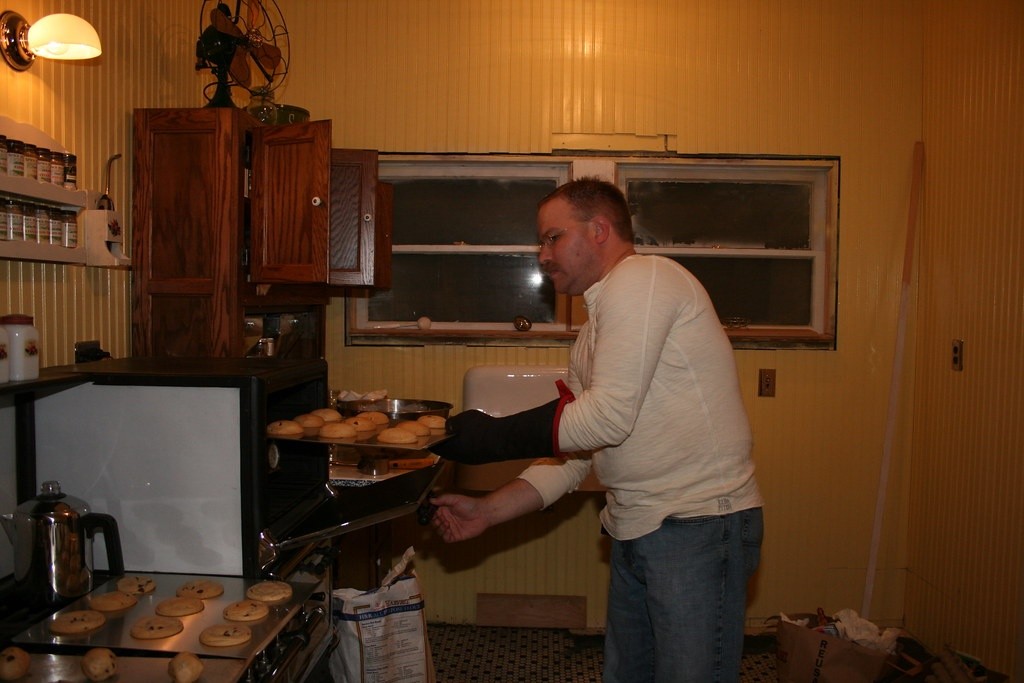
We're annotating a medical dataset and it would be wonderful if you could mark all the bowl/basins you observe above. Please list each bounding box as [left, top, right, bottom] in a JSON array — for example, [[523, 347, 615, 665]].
[[336, 398, 454, 427], [242, 104, 310, 125]]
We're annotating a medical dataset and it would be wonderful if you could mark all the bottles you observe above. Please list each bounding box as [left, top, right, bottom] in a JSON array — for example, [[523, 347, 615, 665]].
[[249, 85, 278, 125], [0, 313, 40, 384]]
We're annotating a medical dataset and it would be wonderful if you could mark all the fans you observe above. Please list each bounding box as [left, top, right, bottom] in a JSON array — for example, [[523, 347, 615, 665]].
[[195, 0, 290, 108]]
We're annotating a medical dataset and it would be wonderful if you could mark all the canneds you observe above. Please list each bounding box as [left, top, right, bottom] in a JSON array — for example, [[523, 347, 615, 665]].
[[0, 197, 78, 248], [0, 135, 77, 191]]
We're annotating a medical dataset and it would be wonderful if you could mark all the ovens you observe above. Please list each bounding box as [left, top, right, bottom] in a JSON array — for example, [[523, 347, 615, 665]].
[[14, 357, 447, 577]]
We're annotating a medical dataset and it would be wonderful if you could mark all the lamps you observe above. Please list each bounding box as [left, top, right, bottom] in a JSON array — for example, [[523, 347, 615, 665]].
[[0, 8, 102, 71]]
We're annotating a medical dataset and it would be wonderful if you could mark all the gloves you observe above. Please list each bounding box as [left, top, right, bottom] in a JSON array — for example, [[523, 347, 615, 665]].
[[428, 379, 576, 467]]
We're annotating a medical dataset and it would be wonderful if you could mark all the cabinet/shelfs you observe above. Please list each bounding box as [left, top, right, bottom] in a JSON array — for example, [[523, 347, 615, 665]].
[[132, 104, 393, 359], [0, 117, 85, 265]]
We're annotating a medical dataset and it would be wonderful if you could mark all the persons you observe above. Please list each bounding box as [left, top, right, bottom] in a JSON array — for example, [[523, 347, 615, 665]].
[[430, 175, 764, 683]]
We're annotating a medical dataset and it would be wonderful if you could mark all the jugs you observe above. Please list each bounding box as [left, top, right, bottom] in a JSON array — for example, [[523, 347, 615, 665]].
[[0, 480, 126, 606]]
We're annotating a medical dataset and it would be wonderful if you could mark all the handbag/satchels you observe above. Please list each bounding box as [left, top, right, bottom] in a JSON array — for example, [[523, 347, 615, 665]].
[[762, 611, 888, 683]]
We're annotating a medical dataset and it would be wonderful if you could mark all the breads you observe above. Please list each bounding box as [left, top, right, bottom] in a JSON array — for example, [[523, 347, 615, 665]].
[[265, 407, 448, 445]]
